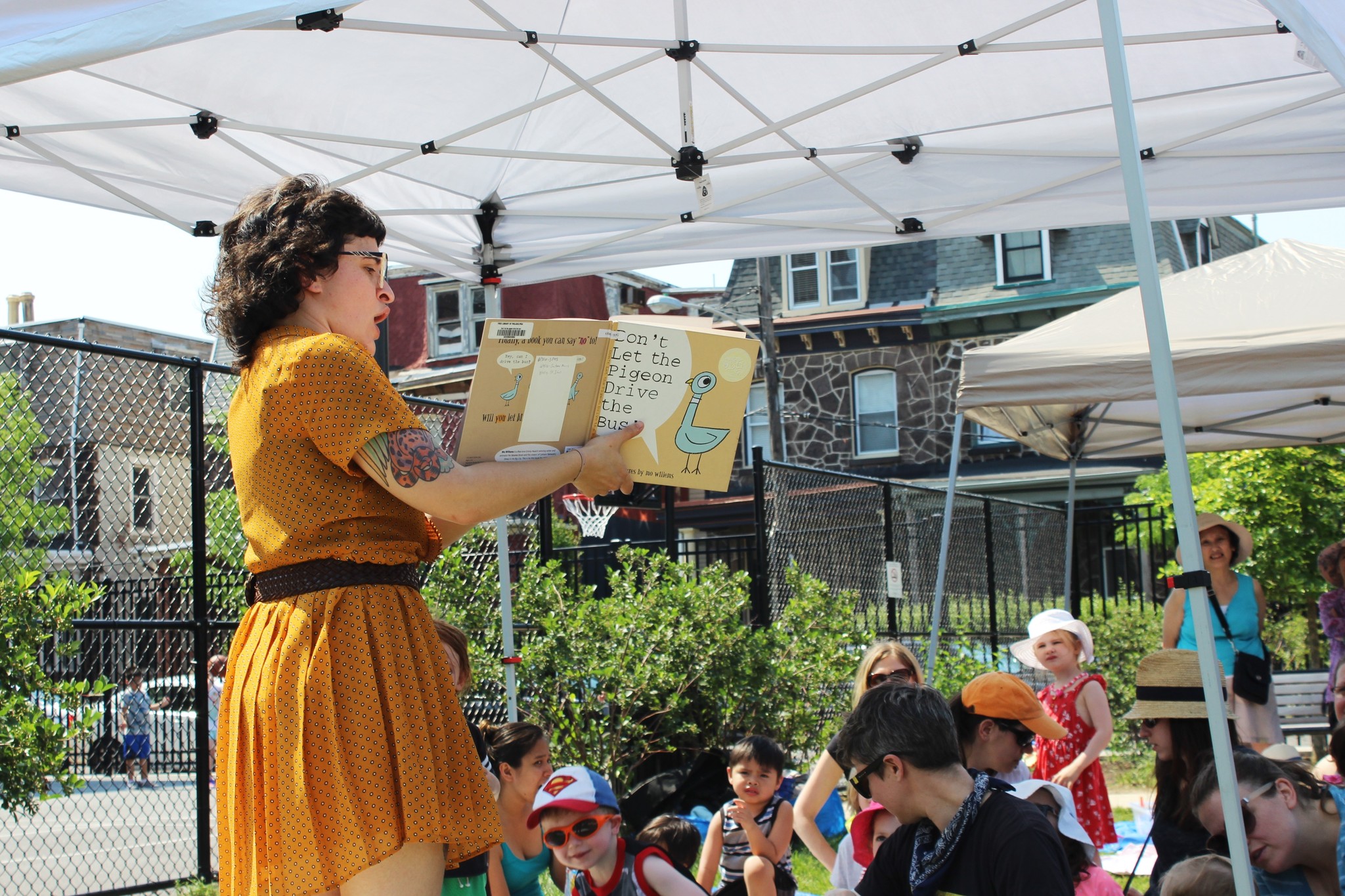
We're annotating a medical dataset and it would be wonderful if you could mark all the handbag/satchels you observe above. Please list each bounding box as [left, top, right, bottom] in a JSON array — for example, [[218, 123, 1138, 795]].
[[1233, 649, 1271, 706]]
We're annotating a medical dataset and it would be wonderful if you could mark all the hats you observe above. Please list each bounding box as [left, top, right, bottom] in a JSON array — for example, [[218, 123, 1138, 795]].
[[850, 800, 885, 869], [1009, 609, 1094, 670], [960, 671, 1069, 740], [526, 765, 620, 830], [1262, 742, 1305, 771], [1175, 512, 1252, 568], [1122, 649, 1240, 720]]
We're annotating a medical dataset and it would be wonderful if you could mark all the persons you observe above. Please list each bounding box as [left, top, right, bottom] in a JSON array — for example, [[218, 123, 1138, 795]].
[[1308, 536, 1345, 788], [1190, 752, 1345, 896], [1160, 511, 1288, 759], [522, 642, 1126, 896], [1118, 648, 1269, 896], [120, 667, 171, 789], [201, 174, 644, 896], [1006, 609, 1119, 872], [207, 670, 225, 791]]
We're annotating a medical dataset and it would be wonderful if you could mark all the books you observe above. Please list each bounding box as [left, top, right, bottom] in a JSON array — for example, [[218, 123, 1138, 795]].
[[455, 314, 761, 492]]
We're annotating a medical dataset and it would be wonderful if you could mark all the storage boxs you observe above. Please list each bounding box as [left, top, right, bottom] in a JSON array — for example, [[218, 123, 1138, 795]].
[[1133, 793, 1154, 835]]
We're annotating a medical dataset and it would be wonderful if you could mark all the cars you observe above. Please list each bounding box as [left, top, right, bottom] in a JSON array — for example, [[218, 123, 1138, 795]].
[[14, 672, 195, 777]]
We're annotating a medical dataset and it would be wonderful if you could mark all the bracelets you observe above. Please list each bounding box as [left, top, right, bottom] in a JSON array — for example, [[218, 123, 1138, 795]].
[[572, 449, 585, 484]]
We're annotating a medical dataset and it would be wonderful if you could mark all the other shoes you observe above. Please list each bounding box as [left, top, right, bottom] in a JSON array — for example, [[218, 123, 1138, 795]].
[[128, 780, 155, 788]]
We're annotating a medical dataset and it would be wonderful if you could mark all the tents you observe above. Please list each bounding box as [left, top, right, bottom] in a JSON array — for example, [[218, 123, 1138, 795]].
[[922, 244, 1345, 700], [0, 0, 1345, 896]]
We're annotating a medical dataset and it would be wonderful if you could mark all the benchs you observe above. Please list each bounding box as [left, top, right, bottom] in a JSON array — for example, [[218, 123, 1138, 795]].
[[1273, 674, 1334, 758]]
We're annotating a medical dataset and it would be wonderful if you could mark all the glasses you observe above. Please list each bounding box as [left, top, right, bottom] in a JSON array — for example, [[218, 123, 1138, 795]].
[[1143, 718, 1159, 728], [332, 252, 387, 289], [1205, 781, 1278, 858], [543, 814, 615, 849], [988, 719, 1035, 748], [867, 668, 914, 688], [848, 751, 911, 799]]
